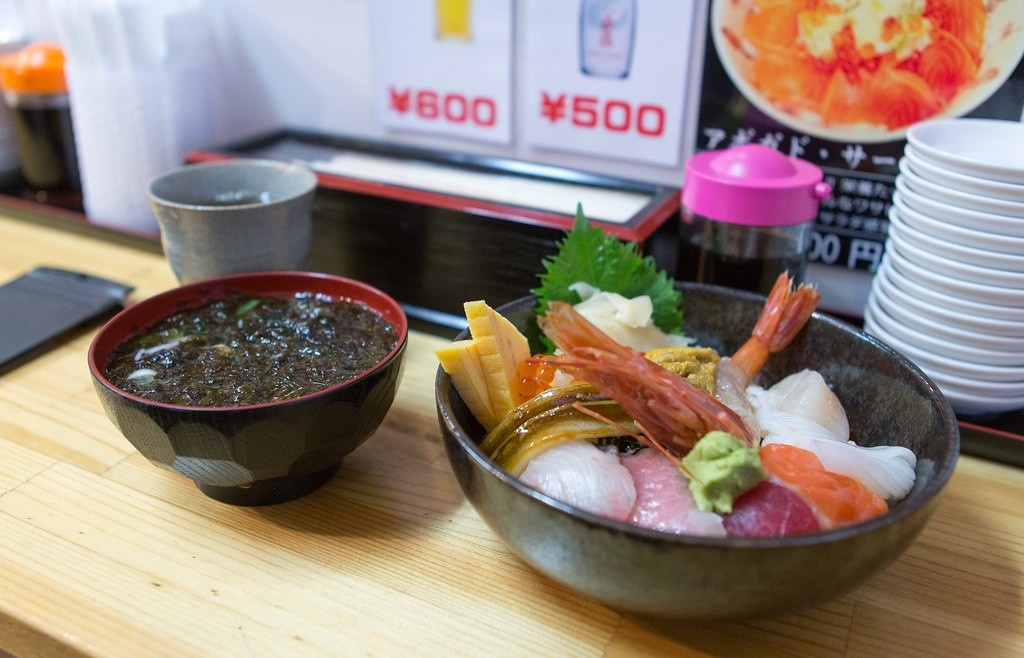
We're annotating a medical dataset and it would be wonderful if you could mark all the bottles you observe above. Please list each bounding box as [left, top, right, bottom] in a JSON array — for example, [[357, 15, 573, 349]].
[[674, 143, 832, 296], [579, 0, 636, 79]]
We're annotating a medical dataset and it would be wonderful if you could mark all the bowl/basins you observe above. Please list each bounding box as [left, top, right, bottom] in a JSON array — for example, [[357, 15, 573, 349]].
[[87, 272, 408, 506], [435, 281, 961, 624]]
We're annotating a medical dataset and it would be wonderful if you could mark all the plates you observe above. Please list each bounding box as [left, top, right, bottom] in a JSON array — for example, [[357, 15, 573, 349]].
[[711, 0, 1023, 144], [863, 119, 1024, 416]]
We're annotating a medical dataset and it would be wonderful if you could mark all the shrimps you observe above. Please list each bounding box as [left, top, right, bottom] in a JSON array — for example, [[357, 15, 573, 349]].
[[526, 269, 820, 457]]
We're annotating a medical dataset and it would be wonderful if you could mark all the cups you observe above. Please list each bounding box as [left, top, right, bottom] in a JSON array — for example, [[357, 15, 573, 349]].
[[145, 159, 319, 285]]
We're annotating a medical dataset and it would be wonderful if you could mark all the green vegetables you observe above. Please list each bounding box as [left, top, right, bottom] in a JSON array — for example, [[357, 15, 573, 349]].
[[526, 207, 685, 353]]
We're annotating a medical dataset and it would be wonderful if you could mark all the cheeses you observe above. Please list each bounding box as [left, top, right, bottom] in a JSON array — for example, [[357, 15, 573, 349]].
[[437, 299, 532, 431]]
